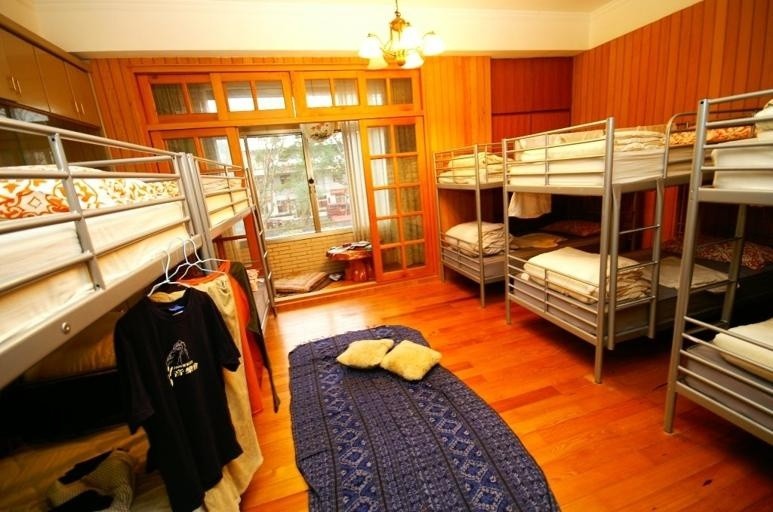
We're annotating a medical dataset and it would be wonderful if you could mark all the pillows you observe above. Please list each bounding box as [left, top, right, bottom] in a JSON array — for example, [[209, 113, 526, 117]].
[[335, 338, 442, 381]]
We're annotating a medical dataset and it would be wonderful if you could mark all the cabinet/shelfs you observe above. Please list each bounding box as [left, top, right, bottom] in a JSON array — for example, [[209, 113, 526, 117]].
[[0, 12, 106, 132]]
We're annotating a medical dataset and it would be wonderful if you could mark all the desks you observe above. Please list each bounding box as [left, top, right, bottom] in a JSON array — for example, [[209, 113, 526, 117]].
[[326, 241, 397, 282]]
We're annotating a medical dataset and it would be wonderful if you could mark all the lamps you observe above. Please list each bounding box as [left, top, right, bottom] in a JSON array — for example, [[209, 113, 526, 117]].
[[360, 0, 445, 69]]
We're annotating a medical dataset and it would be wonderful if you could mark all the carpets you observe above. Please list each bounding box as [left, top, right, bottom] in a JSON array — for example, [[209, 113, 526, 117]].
[[288, 325, 562, 512]]
